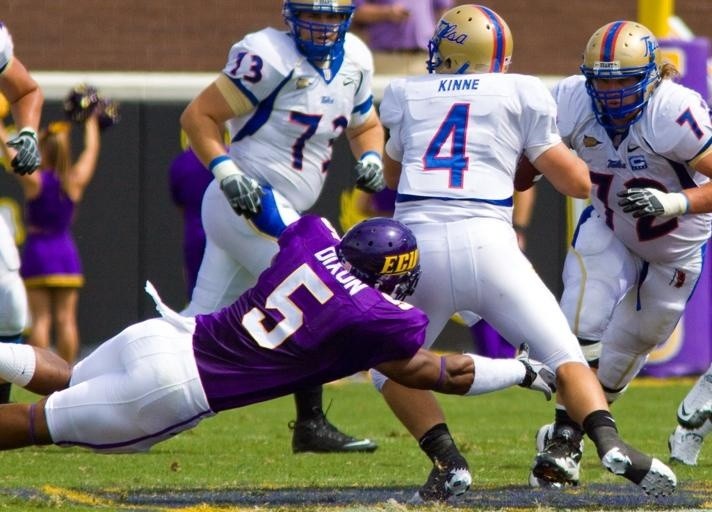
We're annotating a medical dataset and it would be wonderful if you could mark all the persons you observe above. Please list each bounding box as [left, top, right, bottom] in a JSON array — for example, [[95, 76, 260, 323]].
[[352, 0, 454, 55], [0, 24, 45, 407], [1, 186, 476, 451], [168, 126, 233, 306], [668, 363, 712, 467], [180, 2, 380, 453], [370, 4, 678, 505], [514, 20, 712, 490], [1, 113, 100, 369]]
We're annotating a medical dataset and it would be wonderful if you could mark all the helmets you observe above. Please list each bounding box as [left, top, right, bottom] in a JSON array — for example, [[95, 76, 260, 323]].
[[335, 217, 422, 302], [426, 2, 514, 74], [282, 0, 355, 61], [580, 20, 660, 129]]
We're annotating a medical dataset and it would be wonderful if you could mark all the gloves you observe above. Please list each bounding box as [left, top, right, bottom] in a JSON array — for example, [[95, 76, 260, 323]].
[[515, 342, 556, 401], [617, 188, 686, 218], [209, 156, 265, 218], [355, 152, 385, 192], [6, 131, 39, 175]]
